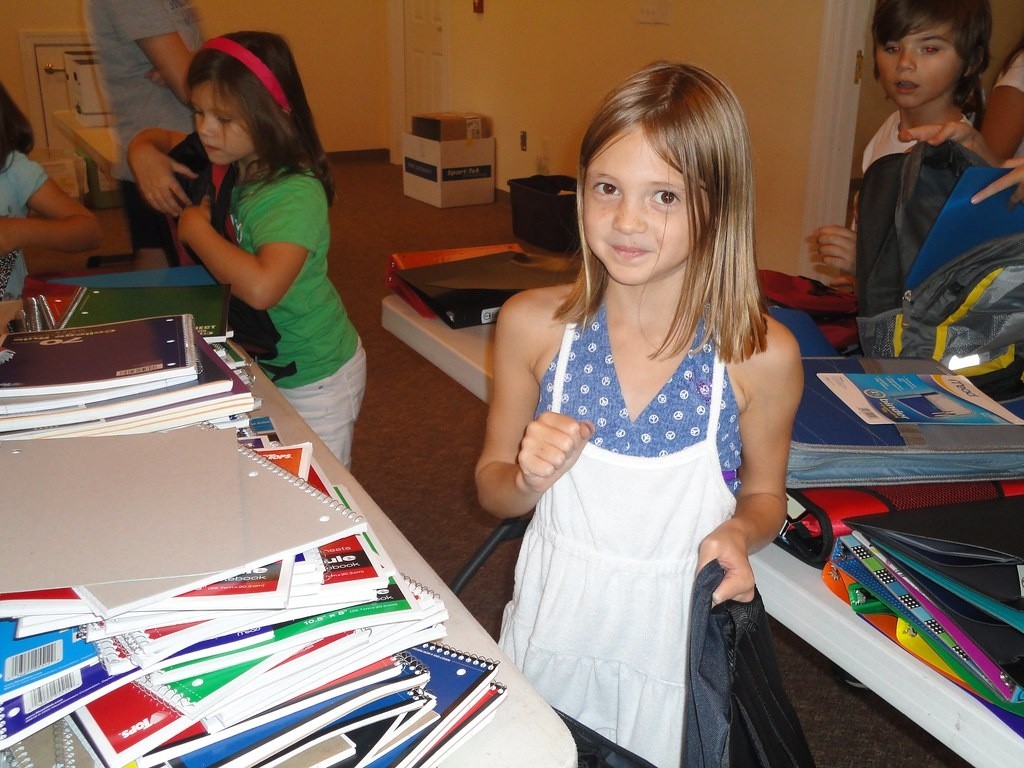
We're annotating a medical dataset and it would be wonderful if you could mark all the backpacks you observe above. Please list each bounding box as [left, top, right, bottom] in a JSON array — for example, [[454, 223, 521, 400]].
[[682, 561, 814, 768], [855, 139, 1024, 379], [163, 131, 298, 382]]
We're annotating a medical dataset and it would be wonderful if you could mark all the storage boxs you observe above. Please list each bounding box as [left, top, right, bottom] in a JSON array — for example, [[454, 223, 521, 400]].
[[408, 109, 494, 145], [62, 49, 118, 127], [400, 129, 496, 209], [506, 176, 580, 252]]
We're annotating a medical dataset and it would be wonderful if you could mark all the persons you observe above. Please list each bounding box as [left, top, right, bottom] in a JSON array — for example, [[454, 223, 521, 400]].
[[475, 60, 804, 768], [895, 39, 1023, 211], [0, 81, 105, 302], [816, 0, 995, 295], [86, 0, 205, 252], [128, 31, 367, 472]]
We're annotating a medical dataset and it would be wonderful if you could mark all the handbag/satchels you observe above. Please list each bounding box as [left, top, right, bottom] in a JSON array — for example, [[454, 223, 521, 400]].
[[757, 268, 859, 357]]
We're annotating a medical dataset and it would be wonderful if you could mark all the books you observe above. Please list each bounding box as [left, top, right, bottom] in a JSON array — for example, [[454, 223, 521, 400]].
[[0, 283, 507, 768]]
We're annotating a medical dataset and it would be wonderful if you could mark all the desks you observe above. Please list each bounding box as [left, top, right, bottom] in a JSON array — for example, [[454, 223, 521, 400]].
[[2, 339, 577, 768], [49, 110, 136, 266], [379, 293, 1024, 768]]
